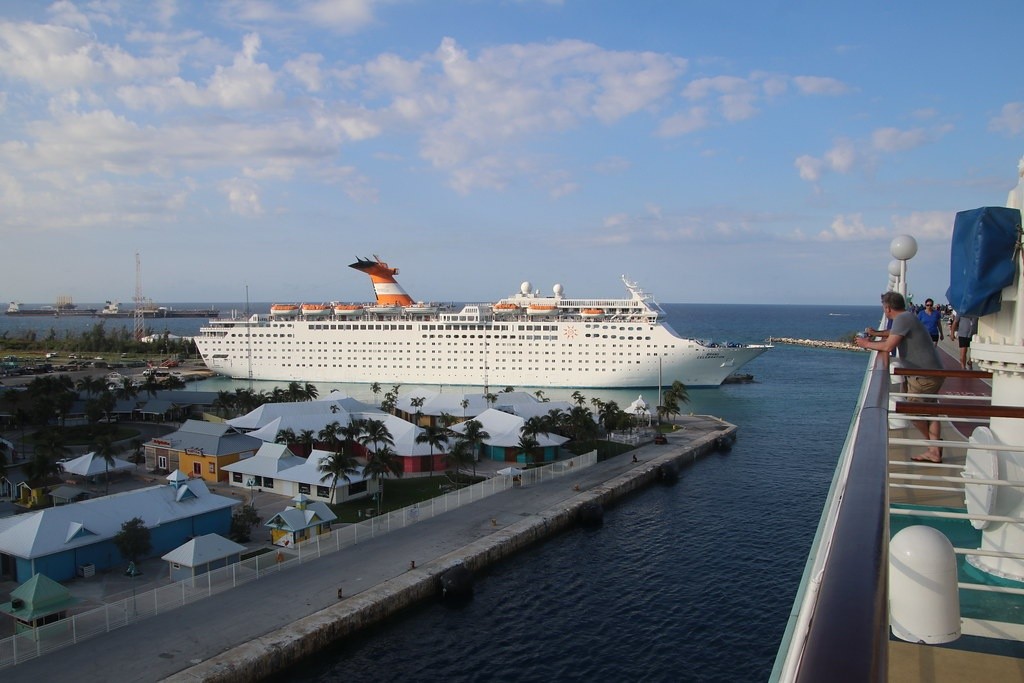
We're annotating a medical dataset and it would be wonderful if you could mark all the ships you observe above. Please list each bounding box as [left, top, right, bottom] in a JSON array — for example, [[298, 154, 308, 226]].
[[190, 253, 775, 389]]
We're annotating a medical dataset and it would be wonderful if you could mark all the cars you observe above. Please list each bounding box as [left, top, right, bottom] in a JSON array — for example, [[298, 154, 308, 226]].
[[59, 352, 133, 370]]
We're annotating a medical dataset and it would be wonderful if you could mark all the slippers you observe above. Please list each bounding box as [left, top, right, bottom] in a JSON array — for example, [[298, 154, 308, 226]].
[[911, 455, 942, 463]]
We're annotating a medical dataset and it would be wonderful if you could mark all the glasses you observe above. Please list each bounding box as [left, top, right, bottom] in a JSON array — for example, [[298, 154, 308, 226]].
[[926, 304, 933, 307]]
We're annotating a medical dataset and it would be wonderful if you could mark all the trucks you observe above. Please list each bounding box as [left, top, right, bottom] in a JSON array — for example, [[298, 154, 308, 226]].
[[143, 366, 171, 378]]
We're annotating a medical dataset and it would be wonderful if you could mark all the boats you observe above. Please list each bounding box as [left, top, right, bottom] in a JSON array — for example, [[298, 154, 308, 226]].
[[270, 303, 300, 317], [404, 301, 439, 315], [141, 333, 183, 345], [301, 303, 332, 316], [368, 302, 403, 316], [491, 303, 522, 317], [334, 304, 363, 316], [525, 303, 559, 316], [580, 308, 605, 319]]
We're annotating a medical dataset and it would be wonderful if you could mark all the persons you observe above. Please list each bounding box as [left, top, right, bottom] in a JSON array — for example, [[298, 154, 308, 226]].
[[633, 454, 637, 461], [854, 292, 948, 463], [909, 298, 978, 370]]
